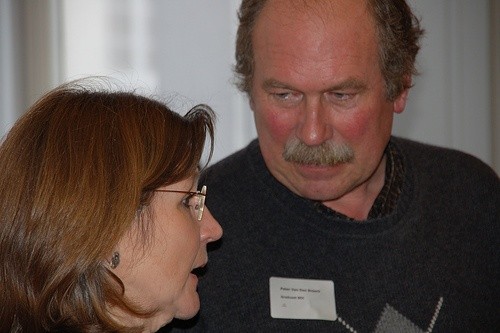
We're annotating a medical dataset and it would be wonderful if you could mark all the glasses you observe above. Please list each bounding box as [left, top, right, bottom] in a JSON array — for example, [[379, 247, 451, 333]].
[[148, 186, 207, 222]]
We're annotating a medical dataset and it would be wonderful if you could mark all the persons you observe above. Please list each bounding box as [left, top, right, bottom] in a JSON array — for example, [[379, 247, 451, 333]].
[[157, 0, 500, 332], [1, 76, 223, 332]]
